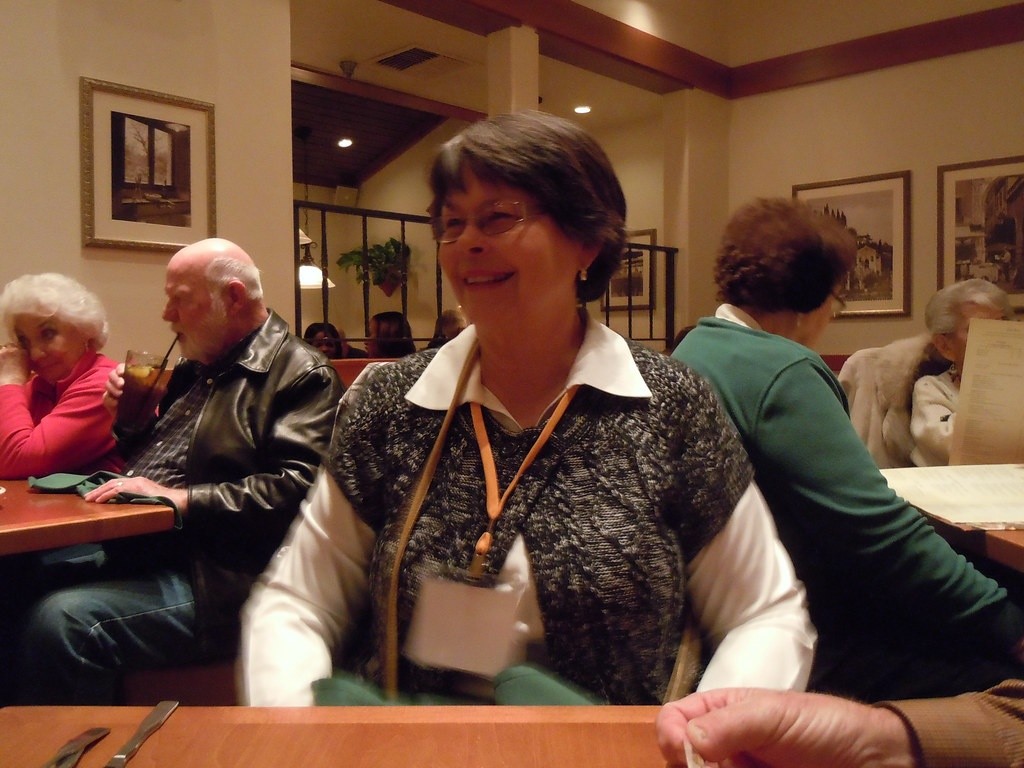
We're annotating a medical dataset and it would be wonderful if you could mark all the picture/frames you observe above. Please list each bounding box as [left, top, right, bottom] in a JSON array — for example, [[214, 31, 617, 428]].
[[936, 155, 1024, 315], [79, 75, 217, 252], [600, 229, 656, 311], [791, 169, 913, 320]]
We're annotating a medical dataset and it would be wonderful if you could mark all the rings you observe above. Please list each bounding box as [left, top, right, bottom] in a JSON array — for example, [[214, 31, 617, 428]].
[[2, 346, 6, 349], [118, 481, 123, 486]]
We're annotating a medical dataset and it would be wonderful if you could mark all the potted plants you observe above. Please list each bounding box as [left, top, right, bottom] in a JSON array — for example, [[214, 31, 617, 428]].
[[336, 238, 411, 298]]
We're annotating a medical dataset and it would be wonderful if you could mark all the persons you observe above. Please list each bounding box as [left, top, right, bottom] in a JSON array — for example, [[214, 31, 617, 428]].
[[241, 109, 817, 704], [659, 679, 1023, 768], [302, 322, 343, 359], [365, 310, 418, 358], [1, 271, 131, 479], [907, 278, 1024, 464], [0, 238, 346, 705], [663, 196, 1024, 701], [337, 326, 369, 358], [427, 309, 468, 349]]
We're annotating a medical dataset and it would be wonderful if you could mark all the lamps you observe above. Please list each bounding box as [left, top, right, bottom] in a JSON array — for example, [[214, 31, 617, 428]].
[[297, 124, 337, 289]]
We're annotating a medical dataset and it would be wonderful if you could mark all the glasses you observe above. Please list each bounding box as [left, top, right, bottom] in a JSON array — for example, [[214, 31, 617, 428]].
[[430, 197, 543, 244], [826, 284, 849, 319]]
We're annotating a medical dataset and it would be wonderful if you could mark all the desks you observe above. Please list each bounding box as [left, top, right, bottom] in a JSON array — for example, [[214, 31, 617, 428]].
[[0, 705, 676, 767], [0, 476, 174, 557]]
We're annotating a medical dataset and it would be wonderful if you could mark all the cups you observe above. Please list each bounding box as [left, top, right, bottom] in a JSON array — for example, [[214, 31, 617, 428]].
[[113, 350, 177, 445]]
